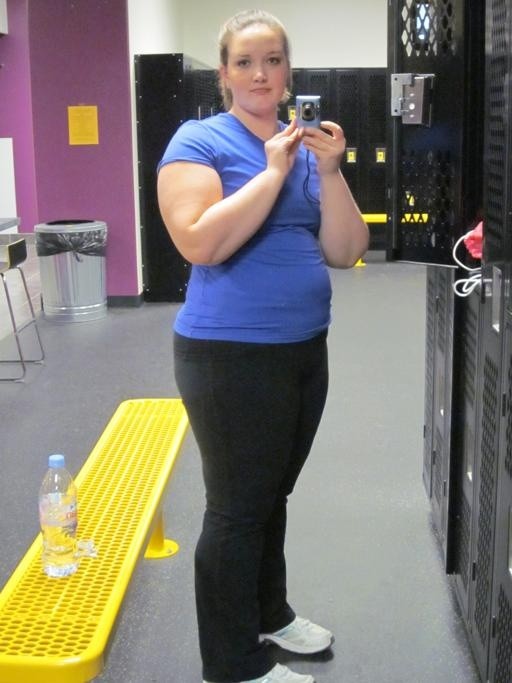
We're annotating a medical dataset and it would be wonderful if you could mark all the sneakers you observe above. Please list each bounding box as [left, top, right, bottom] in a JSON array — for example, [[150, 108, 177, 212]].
[[202, 661, 315, 682], [259, 615, 336, 653]]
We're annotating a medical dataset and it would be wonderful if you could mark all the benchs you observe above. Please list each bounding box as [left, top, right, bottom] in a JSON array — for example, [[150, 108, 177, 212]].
[[1, 396, 191, 682]]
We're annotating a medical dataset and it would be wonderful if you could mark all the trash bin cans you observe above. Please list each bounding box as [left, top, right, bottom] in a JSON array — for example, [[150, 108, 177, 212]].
[[33, 219, 107, 323]]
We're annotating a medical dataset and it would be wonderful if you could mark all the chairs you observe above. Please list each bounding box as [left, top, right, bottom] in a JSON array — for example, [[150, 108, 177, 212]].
[[0, 236, 45, 383]]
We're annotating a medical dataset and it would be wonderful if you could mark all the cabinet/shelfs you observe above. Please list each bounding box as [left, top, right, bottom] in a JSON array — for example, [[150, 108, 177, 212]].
[[134, 53, 390, 304], [377, 1, 512, 683]]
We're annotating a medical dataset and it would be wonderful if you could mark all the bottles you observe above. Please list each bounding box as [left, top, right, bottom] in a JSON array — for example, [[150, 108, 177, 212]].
[[37, 453, 78, 583]]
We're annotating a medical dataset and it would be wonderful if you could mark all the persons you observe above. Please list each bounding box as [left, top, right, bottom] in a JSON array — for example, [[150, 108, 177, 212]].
[[157, 10, 373, 682]]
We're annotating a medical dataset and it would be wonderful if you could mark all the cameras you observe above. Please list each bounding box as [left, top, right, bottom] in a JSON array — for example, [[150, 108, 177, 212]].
[[295, 94, 321, 134]]
